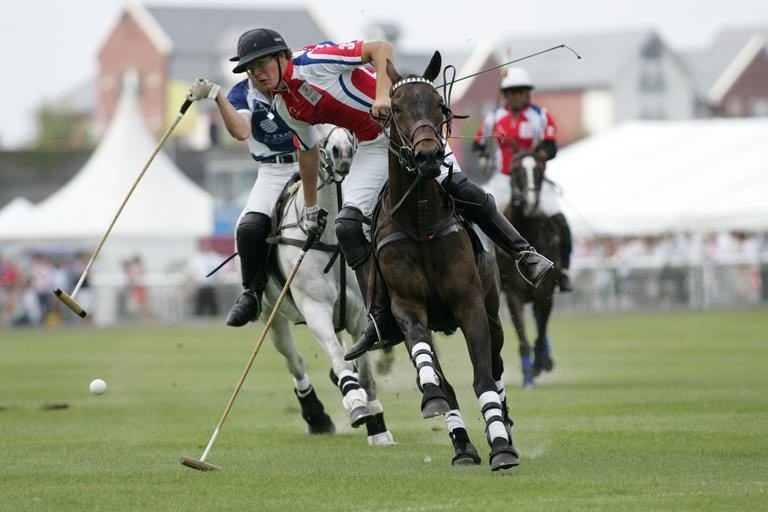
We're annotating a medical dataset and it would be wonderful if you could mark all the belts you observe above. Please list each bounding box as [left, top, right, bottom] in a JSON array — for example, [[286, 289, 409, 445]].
[[261, 152, 298, 163]]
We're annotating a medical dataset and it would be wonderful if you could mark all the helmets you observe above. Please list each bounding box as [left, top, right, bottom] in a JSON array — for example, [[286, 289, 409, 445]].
[[499, 66, 534, 91], [232, 30, 287, 73], [230, 28, 269, 64]]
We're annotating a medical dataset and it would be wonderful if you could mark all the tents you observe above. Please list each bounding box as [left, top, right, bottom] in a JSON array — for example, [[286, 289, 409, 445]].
[[0, 68, 216, 325]]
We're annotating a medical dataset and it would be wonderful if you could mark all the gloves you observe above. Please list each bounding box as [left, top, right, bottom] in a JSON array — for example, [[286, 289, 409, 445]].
[[301, 206, 327, 242], [187, 77, 221, 102]]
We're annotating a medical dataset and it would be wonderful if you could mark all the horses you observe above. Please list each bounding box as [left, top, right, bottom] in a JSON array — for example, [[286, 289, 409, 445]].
[[370, 51, 520, 471], [494, 136, 564, 392], [234, 123, 395, 444]]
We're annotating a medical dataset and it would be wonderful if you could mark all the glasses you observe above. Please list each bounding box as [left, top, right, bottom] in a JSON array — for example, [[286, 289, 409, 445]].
[[246, 57, 275, 73]]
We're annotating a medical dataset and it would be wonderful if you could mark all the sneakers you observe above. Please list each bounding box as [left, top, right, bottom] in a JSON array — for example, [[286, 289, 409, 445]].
[[527, 249, 550, 283], [343, 311, 394, 361], [558, 275, 574, 293], [226, 291, 261, 326]]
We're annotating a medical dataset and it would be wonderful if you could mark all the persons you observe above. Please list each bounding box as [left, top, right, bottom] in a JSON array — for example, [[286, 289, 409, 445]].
[[1, 237, 224, 332], [467, 63, 577, 297], [231, 28, 557, 363], [570, 225, 765, 308], [184, 27, 302, 330]]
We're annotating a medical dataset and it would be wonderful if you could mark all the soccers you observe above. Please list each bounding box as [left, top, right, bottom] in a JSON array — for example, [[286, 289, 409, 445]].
[[90, 380, 105, 395]]
